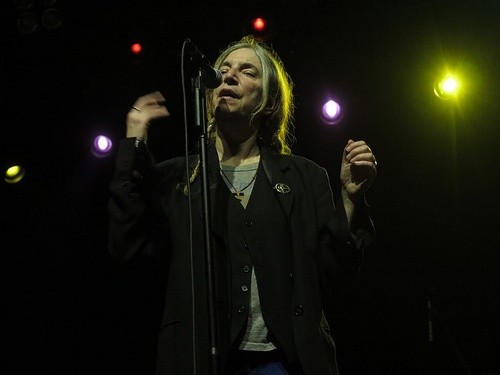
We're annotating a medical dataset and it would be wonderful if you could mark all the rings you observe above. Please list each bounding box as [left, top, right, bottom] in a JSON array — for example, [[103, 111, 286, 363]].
[[373, 161, 378, 168], [131, 104, 141, 113]]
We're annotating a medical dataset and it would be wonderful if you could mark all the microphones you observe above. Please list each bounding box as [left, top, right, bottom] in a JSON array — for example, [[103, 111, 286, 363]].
[[185, 38, 223, 89]]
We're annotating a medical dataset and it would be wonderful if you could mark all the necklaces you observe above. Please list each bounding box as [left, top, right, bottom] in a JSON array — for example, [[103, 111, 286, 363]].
[[218, 167, 258, 200]]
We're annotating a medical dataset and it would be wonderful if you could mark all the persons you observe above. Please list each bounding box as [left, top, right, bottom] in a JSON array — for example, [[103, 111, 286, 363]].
[[105, 37, 380, 375]]
